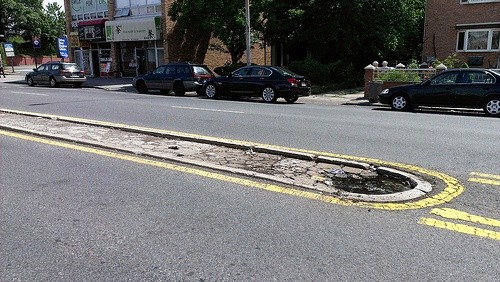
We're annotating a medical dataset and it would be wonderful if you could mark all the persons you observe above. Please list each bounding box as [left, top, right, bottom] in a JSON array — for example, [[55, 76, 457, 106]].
[[0, 58, 6, 78]]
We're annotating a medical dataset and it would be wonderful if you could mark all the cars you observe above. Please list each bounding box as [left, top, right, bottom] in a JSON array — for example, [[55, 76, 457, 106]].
[[131, 63, 221, 96], [378, 67, 500, 118], [26, 61, 87, 88], [195, 64, 312, 103]]
